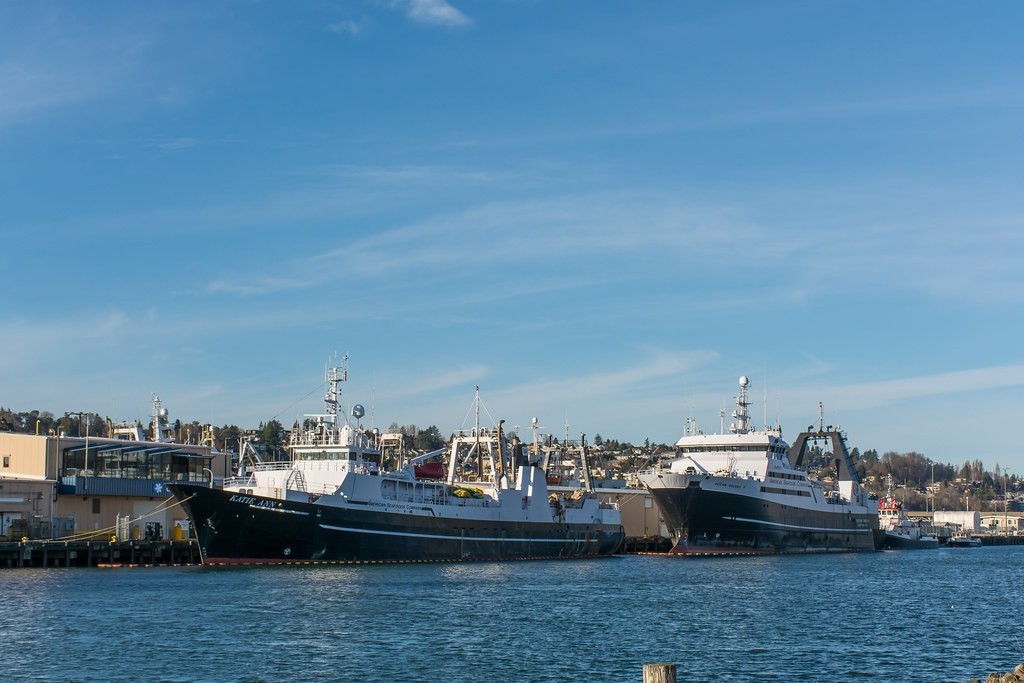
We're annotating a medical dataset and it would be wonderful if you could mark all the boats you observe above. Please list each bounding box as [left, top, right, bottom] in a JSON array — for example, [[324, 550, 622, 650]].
[[882, 525, 940, 550], [945, 534, 983, 548], [636, 363, 886, 555], [159, 349, 631, 568]]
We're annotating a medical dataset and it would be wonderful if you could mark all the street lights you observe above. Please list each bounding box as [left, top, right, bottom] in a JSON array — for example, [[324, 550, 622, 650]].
[[1000, 465, 1012, 537], [928, 460, 938, 525]]
